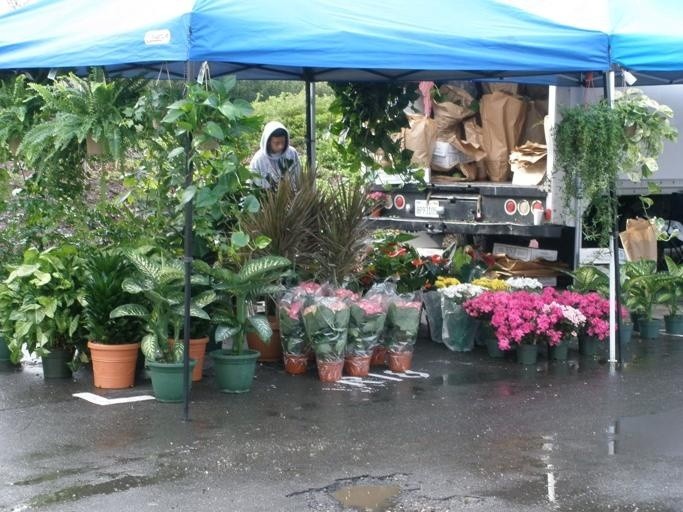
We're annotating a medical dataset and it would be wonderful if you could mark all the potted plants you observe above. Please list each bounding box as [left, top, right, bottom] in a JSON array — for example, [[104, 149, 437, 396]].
[[0, 68, 283, 403], [554, 86, 677, 242], [559, 255, 683, 339]]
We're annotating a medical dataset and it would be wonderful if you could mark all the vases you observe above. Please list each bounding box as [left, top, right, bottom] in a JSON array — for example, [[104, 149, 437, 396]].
[[283, 345, 413, 381], [486, 322, 634, 366]]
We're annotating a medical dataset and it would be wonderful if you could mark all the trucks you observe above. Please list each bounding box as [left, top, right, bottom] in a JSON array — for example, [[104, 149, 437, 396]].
[[352, 80, 683, 286]]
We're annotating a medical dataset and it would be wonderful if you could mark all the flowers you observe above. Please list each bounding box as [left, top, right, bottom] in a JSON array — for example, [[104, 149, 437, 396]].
[[283, 234, 629, 351], [417, 80, 479, 118]]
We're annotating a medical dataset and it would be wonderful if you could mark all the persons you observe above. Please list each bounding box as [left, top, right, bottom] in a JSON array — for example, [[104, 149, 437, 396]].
[[245, 121, 303, 197]]
